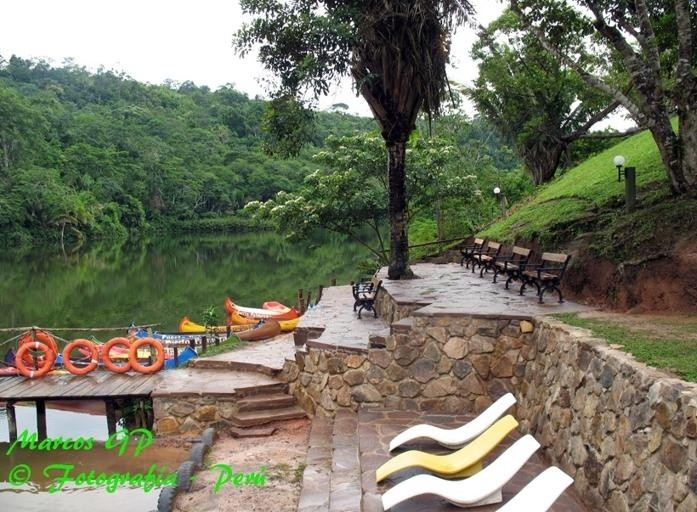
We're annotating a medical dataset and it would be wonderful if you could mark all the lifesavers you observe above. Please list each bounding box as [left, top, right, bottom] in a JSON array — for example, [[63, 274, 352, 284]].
[[15, 332, 57, 377], [63, 338, 99, 374], [130, 337, 165, 373], [103, 338, 132, 373]]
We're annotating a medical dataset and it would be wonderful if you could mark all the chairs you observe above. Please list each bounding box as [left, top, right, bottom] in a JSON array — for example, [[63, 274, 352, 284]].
[[460, 238, 571, 303], [352, 276, 383, 319], [376, 392, 574, 512]]
